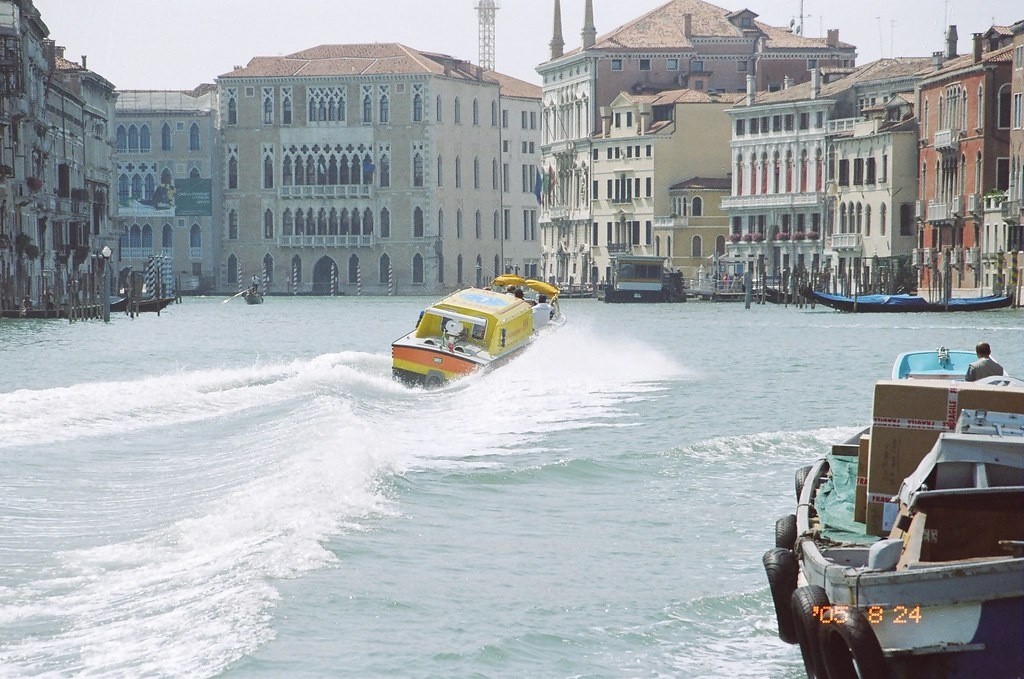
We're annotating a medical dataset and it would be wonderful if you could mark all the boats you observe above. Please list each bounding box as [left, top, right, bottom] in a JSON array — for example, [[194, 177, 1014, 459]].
[[762, 346, 1024, 677], [605, 253, 671, 306], [391, 273, 566, 389], [243, 288, 266, 304], [2, 297, 129, 319], [811, 290, 1014, 313], [132, 296, 174, 316]]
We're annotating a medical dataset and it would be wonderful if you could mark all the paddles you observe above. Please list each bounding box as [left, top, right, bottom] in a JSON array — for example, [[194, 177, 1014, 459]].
[[222, 287, 254, 304]]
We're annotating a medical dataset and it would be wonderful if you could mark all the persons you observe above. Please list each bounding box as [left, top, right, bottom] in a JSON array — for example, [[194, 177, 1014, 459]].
[[663, 265, 681, 274], [698, 267, 757, 294], [964, 341, 1003, 385], [250, 274, 263, 293], [483, 284, 557, 333]]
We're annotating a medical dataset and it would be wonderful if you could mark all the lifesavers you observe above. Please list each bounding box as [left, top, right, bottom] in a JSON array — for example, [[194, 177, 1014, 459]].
[[819, 604, 891, 679], [790, 584, 831, 679], [762, 548, 800, 644], [775, 515, 796, 550], [795, 466, 820, 517]]
